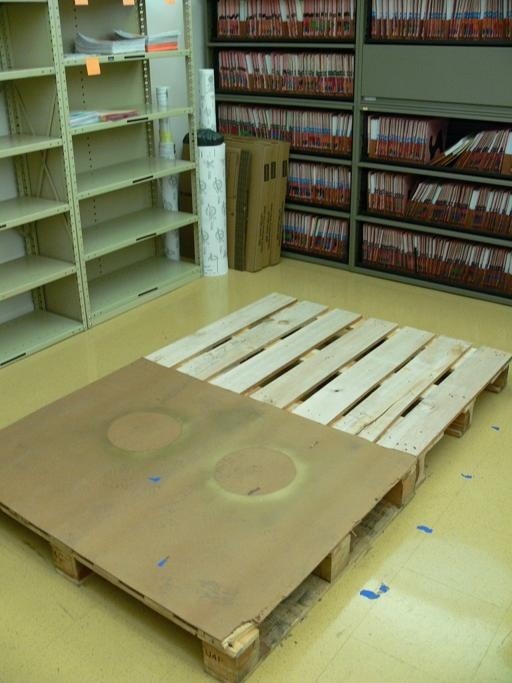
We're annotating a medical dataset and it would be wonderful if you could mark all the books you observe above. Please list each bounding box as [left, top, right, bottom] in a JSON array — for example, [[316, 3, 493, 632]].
[[368, 170, 512, 237], [364, 224, 512, 291], [289, 160, 351, 212], [218, 50, 354, 97], [371, 0, 512, 42], [283, 210, 348, 258], [65, 23, 183, 129], [217, 1, 356, 41], [367, 114, 512, 176], [219, 105, 352, 156]]
